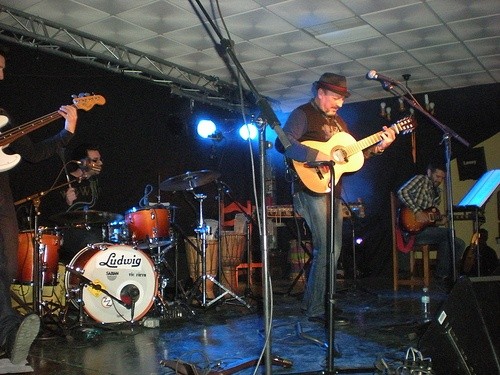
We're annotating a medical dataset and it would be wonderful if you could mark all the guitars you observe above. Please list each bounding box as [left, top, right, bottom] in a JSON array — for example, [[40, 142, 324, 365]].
[[396, 205, 486, 235], [0, 91, 107, 172], [285, 115, 419, 199]]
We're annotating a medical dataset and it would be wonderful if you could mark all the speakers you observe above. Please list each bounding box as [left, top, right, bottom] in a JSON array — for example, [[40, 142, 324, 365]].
[[417, 275, 499, 375]]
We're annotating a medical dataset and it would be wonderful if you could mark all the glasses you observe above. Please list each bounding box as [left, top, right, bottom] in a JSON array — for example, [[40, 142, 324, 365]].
[[91, 158, 104, 163]]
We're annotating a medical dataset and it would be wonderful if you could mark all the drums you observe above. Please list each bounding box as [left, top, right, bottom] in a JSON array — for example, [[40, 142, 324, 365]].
[[124, 202, 175, 250], [64, 242, 159, 328], [12, 228, 61, 287], [183, 235, 217, 300], [106, 221, 128, 243], [216, 230, 247, 298]]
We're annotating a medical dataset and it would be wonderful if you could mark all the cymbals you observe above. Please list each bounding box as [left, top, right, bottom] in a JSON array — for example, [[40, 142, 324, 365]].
[[46, 209, 123, 225], [159, 170, 220, 191]]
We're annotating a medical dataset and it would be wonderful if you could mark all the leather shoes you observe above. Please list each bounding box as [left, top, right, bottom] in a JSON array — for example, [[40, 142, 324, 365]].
[[302, 307, 350, 326]]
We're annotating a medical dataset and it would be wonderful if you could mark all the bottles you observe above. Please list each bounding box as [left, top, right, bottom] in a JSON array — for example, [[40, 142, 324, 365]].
[[421, 287, 432, 323]]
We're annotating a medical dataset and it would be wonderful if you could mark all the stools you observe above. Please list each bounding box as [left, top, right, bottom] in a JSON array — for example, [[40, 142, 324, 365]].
[[236, 262, 263, 289]]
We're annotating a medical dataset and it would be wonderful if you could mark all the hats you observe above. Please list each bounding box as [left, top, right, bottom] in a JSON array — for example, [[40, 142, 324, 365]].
[[313, 72, 350, 97]]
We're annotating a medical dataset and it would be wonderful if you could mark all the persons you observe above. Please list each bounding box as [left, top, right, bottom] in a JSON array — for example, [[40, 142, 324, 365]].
[[275, 73, 395, 327], [398, 159, 467, 282], [0, 52, 118, 365]]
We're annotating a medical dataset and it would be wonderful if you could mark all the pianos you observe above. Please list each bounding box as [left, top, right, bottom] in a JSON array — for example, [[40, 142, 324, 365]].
[[265, 202, 366, 297]]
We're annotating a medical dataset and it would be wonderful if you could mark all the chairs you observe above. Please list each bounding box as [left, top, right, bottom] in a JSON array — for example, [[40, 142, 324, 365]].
[[390, 190, 439, 292], [220, 199, 256, 276]]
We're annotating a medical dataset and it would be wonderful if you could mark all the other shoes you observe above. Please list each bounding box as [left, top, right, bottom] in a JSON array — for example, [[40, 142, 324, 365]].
[[7, 313, 40, 365]]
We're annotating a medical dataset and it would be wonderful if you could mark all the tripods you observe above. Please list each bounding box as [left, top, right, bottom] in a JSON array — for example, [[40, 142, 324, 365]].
[[15, 171, 131, 342], [157, 177, 266, 321]]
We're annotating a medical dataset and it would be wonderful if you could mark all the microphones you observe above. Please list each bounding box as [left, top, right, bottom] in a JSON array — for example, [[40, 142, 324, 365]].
[[74, 161, 101, 171], [368, 70, 401, 84], [208, 134, 225, 139], [130, 288, 138, 320]]
